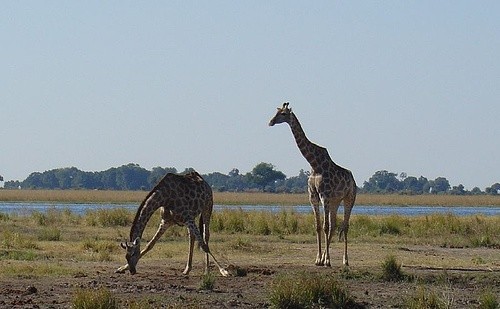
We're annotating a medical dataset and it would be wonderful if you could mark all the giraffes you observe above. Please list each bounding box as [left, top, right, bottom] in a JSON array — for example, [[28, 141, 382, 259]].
[[269, 102, 357, 267], [113, 171, 232, 278]]
